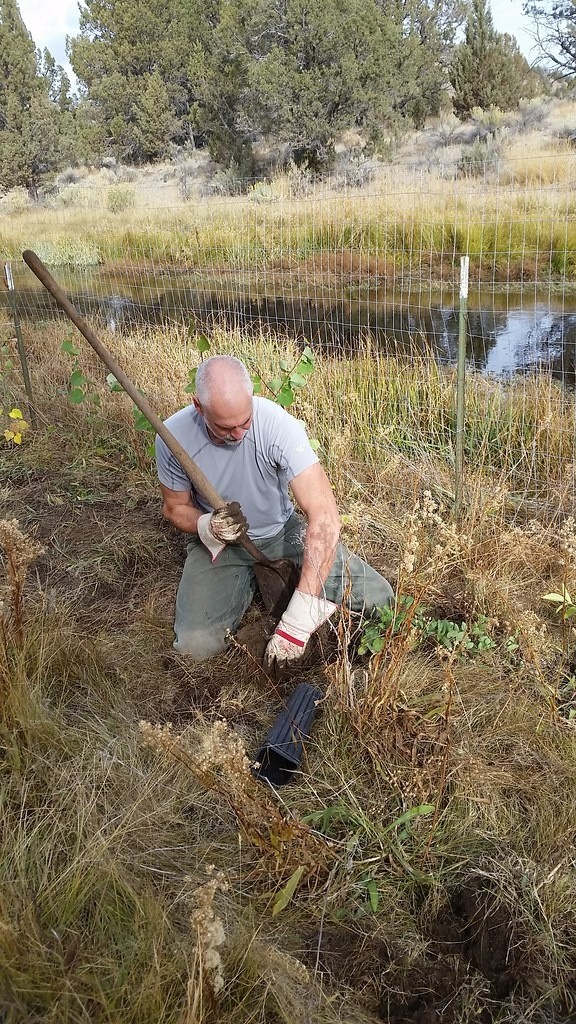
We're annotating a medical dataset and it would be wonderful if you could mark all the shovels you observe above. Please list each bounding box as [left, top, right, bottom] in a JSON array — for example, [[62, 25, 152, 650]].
[[21, 249, 302, 622]]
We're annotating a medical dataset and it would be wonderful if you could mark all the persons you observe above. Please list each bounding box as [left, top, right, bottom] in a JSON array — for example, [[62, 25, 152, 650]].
[[155, 357, 394, 675]]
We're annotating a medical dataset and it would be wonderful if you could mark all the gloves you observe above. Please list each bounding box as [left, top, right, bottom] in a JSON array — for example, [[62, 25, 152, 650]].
[[197, 501, 250, 565], [261, 587, 338, 671]]
